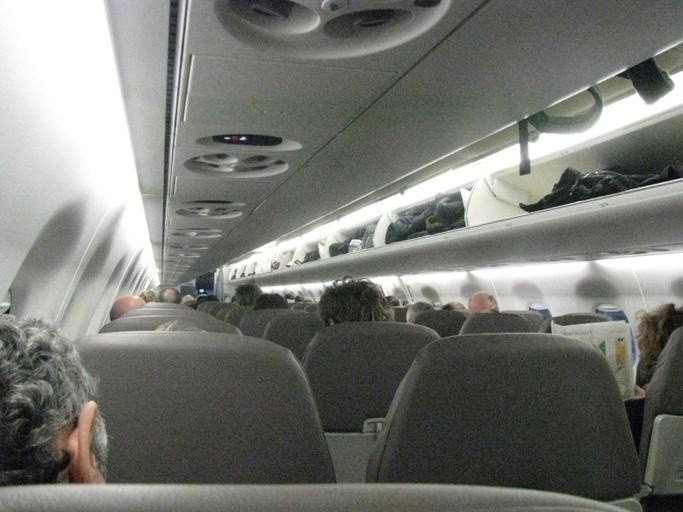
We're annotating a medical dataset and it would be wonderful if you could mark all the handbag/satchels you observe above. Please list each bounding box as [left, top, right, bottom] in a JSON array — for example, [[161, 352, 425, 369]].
[[304, 249, 320, 262], [329, 224, 373, 255], [386, 197, 465, 243]]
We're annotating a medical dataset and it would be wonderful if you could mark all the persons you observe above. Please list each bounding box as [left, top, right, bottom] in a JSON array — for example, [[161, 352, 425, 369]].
[[387, 292, 681, 456], [109, 277, 392, 327], [0, 314, 107, 485]]
